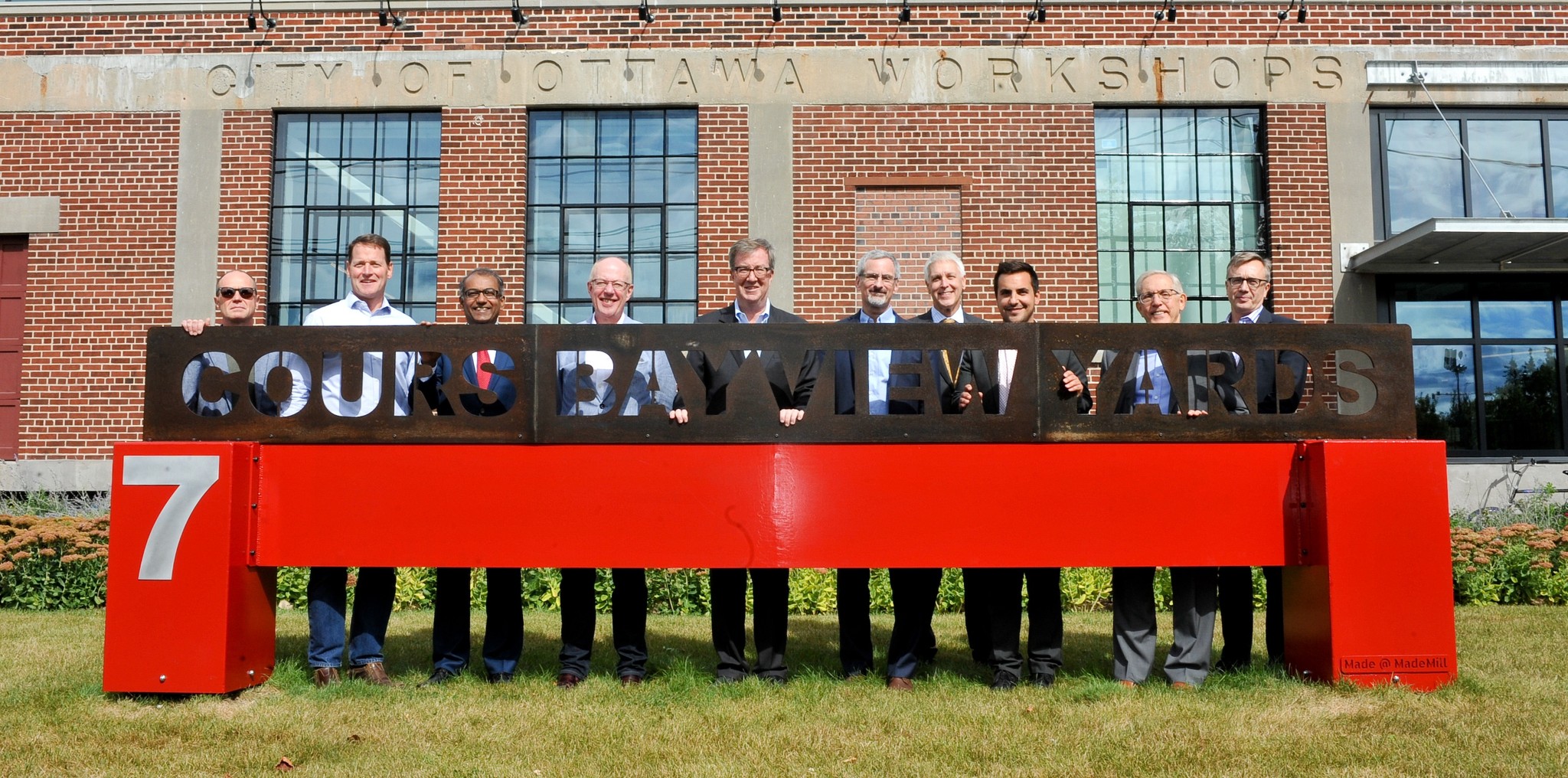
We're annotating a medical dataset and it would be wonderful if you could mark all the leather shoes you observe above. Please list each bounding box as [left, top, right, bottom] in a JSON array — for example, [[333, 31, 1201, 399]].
[[490, 672, 512, 684], [990, 670, 1018, 692], [416, 667, 454, 688], [888, 676, 913, 692], [314, 667, 341, 690], [348, 661, 404, 688], [848, 674, 866, 684], [557, 673, 581, 690], [620, 675, 641, 688], [1033, 672, 1055, 690]]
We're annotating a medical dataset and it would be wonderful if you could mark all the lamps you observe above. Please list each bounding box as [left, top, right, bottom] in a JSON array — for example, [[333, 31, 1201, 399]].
[[1277, 0, 1307, 23], [378, 1, 403, 26], [772, 0, 783, 22], [1154, 0, 1176, 22], [1028, 0, 1046, 23], [898, 0, 911, 23], [511, 0, 528, 25], [638, 0, 655, 23], [247, 0, 277, 31]]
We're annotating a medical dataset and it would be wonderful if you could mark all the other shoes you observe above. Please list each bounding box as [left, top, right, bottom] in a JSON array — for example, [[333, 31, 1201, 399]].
[[764, 676, 783, 687], [712, 676, 735, 686], [1117, 680, 1136, 689], [1214, 661, 1250, 674], [1172, 681, 1195, 691]]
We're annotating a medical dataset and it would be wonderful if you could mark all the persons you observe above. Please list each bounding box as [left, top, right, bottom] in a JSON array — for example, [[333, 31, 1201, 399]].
[[669, 238, 818, 683], [825, 251, 1093, 695], [411, 268, 531, 685], [181, 270, 277, 418], [270, 234, 419, 689], [550, 256, 676, 686], [1096, 270, 1249, 693], [1214, 252, 1307, 678]]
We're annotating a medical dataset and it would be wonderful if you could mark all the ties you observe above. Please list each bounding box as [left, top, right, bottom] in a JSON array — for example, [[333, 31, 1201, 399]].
[[477, 350, 492, 389], [940, 318, 961, 389]]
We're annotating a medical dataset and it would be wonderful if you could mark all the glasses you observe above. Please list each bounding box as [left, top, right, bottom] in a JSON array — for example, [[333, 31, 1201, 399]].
[[1136, 289, 1180, 304], [459, 288, 501, 299], [216, 287, 257, 300], [1228, 276, 1268, 287], [859, 273, 897, 285], [589, 278, 631, 291], [733, 266, 771, 277]]
